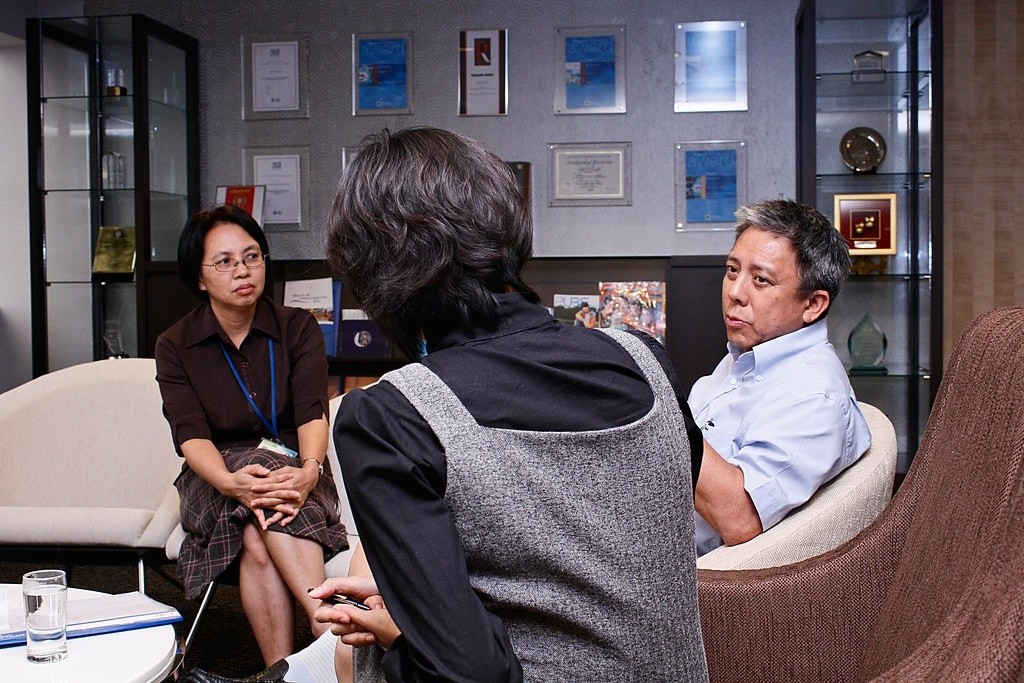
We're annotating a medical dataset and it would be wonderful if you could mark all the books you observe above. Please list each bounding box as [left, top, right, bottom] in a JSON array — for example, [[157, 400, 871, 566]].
[[284, 275, 335, 327]]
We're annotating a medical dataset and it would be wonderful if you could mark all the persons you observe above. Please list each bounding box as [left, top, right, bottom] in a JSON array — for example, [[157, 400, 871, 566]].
[[308, 123, 709, 683], [684, 200, 871, 558], [574, 302, 596, 328], [157, 200, 352, 683]]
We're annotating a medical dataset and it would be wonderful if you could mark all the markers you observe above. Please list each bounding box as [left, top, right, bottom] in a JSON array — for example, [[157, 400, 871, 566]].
[[307, 588, 371, 611]]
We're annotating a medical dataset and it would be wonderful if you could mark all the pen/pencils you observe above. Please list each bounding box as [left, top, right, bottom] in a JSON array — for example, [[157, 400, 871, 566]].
[[700, 421, 715, 430]]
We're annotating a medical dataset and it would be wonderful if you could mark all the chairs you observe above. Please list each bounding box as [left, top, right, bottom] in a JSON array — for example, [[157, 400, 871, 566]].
[[694, 305, 1024, 683], [0, 356, 359, 654]]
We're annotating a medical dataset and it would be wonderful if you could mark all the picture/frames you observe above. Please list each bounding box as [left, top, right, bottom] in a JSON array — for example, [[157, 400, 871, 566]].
[[834, 193, 897, 255]]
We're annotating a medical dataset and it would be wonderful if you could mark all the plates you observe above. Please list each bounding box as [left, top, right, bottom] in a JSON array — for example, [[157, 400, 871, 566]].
[[839, 127, 887, 171]]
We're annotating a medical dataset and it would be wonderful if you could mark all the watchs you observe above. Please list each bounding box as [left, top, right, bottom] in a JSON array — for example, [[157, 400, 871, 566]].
[[301, 458, 324, 479]]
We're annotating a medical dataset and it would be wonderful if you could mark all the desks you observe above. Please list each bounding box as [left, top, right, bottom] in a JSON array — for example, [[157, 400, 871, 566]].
[[0, 584, 179, 683]]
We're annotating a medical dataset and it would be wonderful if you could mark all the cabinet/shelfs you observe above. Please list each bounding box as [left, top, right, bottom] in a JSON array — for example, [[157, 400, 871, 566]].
[[24, 12, 202, 380], [794, 0, 943, 490]]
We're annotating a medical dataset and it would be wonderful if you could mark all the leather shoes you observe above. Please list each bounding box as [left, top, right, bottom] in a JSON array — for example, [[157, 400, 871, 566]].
[[175, 657, 289, 683]]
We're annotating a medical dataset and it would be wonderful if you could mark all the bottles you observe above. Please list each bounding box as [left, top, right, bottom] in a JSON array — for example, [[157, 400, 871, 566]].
[[101, 152, 120, 189]]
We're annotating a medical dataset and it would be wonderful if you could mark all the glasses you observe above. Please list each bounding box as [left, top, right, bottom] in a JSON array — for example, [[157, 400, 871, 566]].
[[202, 252, 269, 272]]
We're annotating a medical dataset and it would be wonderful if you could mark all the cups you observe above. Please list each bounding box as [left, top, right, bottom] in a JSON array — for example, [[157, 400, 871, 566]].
[[23, 569, 68, 663]]
